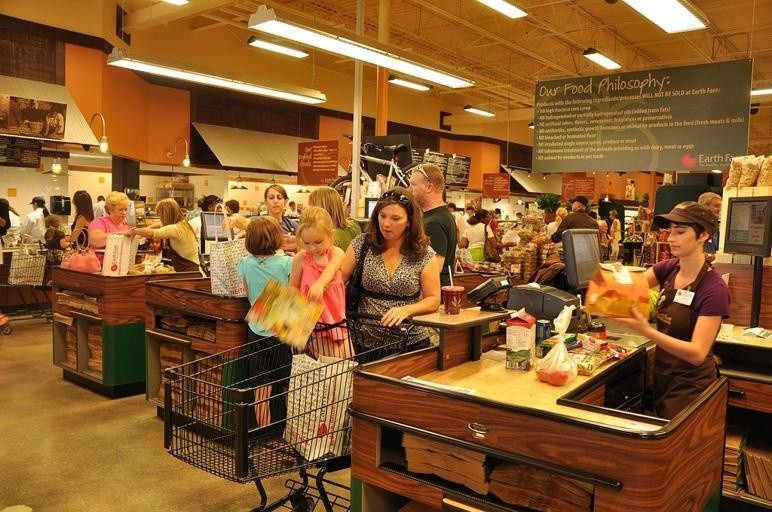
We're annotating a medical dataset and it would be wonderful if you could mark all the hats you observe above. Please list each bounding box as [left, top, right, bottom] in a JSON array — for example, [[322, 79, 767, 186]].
[[568, 196, 588, 207], [29, 197, 45, 205], [653, 202, 718, 238]]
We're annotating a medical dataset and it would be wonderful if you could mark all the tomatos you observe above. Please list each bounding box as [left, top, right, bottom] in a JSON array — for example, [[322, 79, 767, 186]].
[[536, 369, 569, 386]]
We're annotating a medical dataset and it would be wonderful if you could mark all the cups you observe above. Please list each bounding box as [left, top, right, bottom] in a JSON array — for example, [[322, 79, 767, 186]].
[[440, 285, 466, 315]]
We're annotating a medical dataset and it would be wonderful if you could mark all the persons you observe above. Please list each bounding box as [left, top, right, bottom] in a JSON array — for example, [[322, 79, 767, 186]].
[[18, 99, 65, 140]]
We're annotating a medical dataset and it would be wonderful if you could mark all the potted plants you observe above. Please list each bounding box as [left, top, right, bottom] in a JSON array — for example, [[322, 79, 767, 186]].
[[534, 192, 563, 224]]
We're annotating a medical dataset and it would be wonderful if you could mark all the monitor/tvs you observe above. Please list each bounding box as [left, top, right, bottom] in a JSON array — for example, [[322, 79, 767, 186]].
[[365, 198, 380, 218], [562, 228, 603, 290], [723, 197, 772, 257], [201, 211, 234, 241]]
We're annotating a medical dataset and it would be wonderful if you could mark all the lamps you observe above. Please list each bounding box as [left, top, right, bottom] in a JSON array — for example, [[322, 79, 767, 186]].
[[82, 112, 108, 153], [464, 105, 494, 117], [582, 41, 622, 70], [246, 4, 476, 92], [751, 89, 771, 96], [296, 184, 311, 193], [528, 123, 536, 130], [478, 0, 528, 20], [711, 170, 723, 173], [624, 1, 711, 35], [166, 136, 191, 167], [230, 171, 247, 190], [601, 196, 611, 201], [41, 143, 72, 176], [106, 48, 327, 106]]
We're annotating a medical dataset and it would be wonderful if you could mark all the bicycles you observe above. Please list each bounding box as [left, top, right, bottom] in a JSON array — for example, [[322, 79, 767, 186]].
[[328, 133, 436, 216]]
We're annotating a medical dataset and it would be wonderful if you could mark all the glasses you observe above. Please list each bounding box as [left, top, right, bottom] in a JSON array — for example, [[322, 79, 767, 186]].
[[379, 192, 414, 209], [415, 163, 431, 184]]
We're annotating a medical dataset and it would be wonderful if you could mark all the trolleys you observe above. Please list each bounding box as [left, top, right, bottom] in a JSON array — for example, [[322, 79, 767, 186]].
[[0, 240, 60, 335], [156, 309, 434, 511]]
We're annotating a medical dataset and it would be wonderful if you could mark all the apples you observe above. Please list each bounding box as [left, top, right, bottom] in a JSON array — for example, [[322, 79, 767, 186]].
[[680, 153, 698, 169], [111, 265, 117, 271], [317, 423, 327, 438]]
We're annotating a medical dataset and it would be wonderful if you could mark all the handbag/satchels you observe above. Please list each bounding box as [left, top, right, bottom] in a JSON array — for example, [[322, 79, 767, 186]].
[[7, 233, 46, 288], [484, 224, 502, 262], [282, 350, 359, 460], [344, 232, 371, 318], [209, 202, 252, 299], [59, 228, 102, 273]]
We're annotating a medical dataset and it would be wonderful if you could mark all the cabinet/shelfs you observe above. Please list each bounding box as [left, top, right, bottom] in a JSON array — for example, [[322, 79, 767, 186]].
[[596, 195, 647, 251]]
